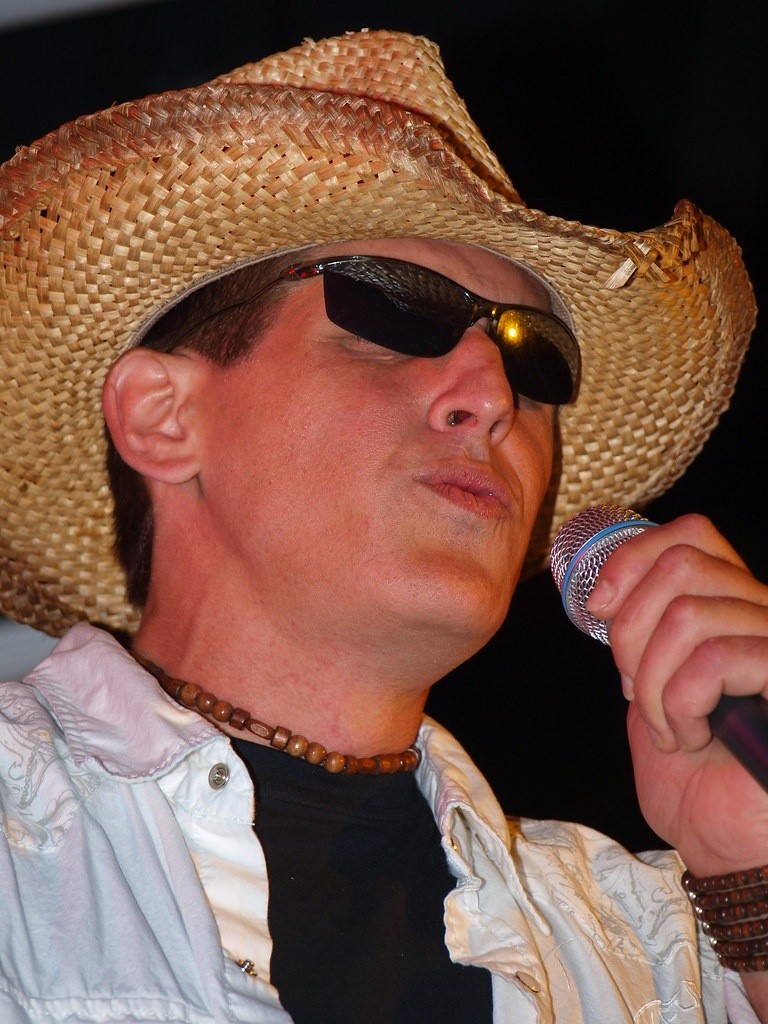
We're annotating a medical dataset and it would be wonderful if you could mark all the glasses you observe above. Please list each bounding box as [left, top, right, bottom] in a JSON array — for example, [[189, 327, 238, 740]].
[[141, 252, 583, 406]]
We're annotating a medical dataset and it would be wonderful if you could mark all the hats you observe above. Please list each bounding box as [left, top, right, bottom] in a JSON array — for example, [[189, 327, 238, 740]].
[[0, 30, 760, 642]]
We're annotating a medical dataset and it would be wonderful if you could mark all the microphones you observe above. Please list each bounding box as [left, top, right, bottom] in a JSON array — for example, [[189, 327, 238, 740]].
[[550, 503, 768, 795]]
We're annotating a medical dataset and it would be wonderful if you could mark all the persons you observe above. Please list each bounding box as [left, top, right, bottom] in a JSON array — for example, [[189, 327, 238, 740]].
[[0, 27, 768, 1022]]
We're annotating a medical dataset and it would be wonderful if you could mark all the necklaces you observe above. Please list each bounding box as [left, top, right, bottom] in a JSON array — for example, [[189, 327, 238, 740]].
[[125, 652, 423, 779]]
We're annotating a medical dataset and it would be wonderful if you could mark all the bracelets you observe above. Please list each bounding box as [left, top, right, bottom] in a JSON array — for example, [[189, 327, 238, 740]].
[[682, 866, 767, 974]]
